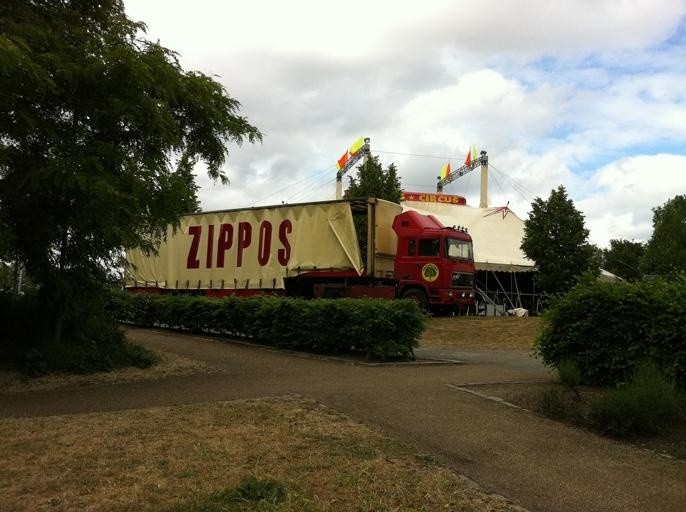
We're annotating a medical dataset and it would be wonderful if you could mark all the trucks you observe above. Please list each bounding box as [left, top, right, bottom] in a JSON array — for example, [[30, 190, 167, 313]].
[[116, 197, 476, 316]]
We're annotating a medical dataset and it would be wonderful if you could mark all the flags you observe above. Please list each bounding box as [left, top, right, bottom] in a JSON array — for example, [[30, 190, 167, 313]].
[[445, 162, 451, 176], [464, 150, 471, 167], [334, 136, 363, 170], [472, 145, 478, 160], [441, 163, 446, 179]]
